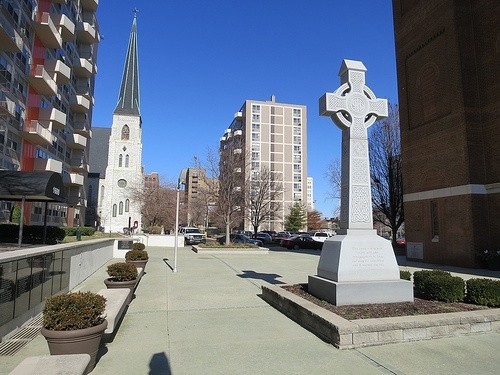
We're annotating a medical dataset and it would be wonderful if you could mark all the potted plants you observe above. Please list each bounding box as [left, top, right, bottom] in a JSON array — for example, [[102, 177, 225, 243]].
[[125, 250, 149, 272], [40, 291, 108, 375], [104, 262, 138, 292]]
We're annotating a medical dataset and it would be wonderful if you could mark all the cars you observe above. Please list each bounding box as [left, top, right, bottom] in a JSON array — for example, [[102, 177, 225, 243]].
[[280, 235, 323, 250], [383, 236, 405, 244], [215, 234, 263, 247], [240, 230, 290, 243]]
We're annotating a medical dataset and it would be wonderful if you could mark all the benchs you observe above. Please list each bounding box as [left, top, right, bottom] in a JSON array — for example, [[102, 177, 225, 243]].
[[96, 288, 132, 334], [7, 354, 91, 375], [134, 266, 144, 288]]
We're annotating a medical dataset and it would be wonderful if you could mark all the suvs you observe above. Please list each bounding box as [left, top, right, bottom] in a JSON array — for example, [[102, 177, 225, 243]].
[[179, 226, 205, 244], [308, 232, 329, 242]]
